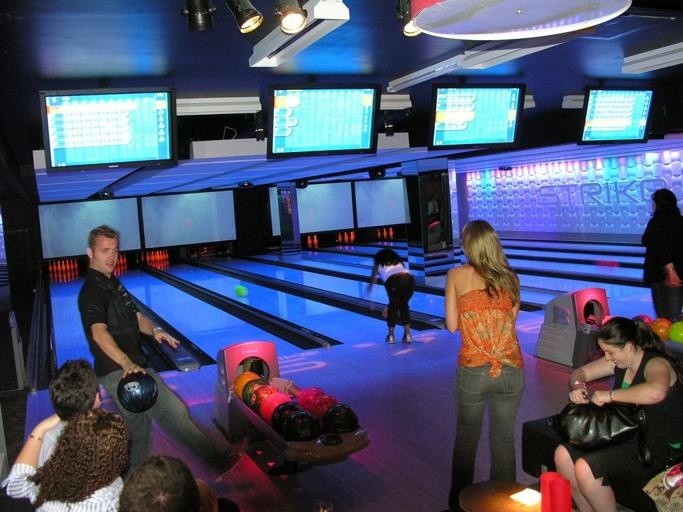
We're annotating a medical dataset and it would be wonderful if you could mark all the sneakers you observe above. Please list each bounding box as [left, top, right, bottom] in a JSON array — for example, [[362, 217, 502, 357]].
[[402, 334, 412, 343], [386, 335, 395, 344]]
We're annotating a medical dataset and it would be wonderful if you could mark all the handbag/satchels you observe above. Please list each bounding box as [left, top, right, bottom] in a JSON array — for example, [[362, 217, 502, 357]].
[[555, 398, 645, 450]]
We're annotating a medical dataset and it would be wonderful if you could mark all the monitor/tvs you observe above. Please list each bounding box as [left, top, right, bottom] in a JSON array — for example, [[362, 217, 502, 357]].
[[265, 85, 382, 160], [39, 87, 180, 174], [426, 85, 529, 151], [577, 85, 657, 145]]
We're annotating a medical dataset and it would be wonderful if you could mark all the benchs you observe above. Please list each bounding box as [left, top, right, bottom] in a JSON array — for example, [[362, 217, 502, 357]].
[[522, 413, 683, 512]]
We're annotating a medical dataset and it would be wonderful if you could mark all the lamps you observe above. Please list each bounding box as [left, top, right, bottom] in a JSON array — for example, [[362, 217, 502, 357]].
[[411, 1, 632, 41], [224, 1, 264, 33], [273, 1, 307, 33], [183, 1, 216, 33], [248, 1, 350, 68], [399, 2, 421, 37]]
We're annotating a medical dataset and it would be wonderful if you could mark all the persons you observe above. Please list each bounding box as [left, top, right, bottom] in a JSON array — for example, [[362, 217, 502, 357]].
[[77, 225, 241, 486], [118, 455, 219, 512], [553, 317, 683, 512], [641, 188, 683, 323], [36, 358, 102, 468], [368, 249, 415, 343], [444, 220, 525, 512], [0, 408, 130, 512]]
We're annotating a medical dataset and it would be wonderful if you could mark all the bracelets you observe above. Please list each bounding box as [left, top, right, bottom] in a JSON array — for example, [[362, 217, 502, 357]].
[[152, 327, 165, 333], [609, 389, 613, 402], [28, 434, 42, 442], [663, 268, 676, 273], [571, 383, 586, 390]]
